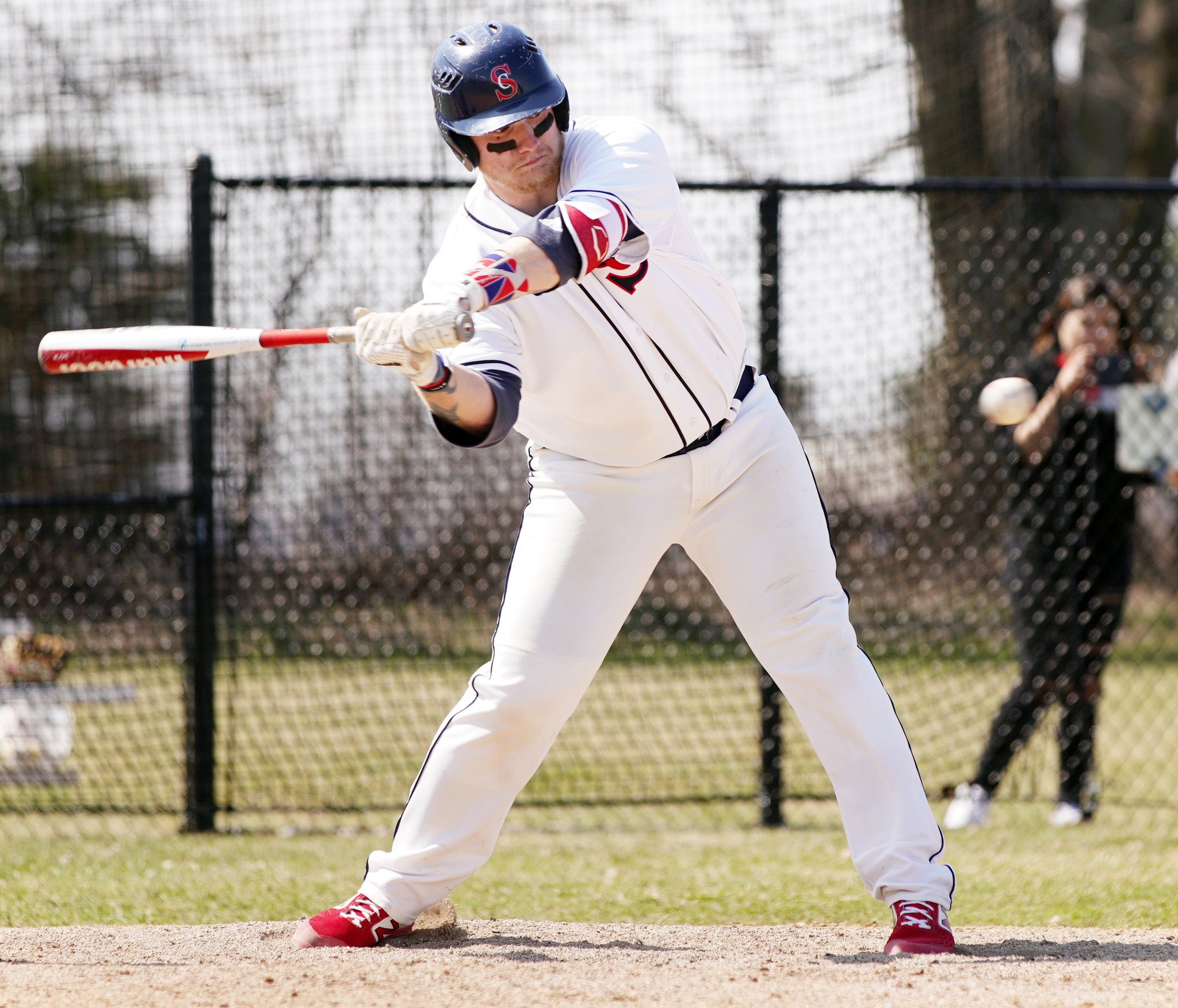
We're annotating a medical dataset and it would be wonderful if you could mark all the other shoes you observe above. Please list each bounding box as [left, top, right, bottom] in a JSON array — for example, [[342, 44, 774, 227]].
[[943, 783, 990, 828], [1049, 803, 1085, 827]]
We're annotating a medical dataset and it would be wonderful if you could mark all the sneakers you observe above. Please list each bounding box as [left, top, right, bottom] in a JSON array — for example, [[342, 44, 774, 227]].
[[885, 898, 956, 955], [293, 891, 413, 950]]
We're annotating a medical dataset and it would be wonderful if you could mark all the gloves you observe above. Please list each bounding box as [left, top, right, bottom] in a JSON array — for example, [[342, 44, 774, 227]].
[[397, 276, 483, 352], [354, 306, 439, 387]]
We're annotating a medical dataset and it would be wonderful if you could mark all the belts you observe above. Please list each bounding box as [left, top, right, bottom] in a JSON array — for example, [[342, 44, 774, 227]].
[[659, 364, 756, 458]]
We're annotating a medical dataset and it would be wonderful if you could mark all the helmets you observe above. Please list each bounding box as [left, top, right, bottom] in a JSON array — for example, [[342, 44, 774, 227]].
[[431, 22, 570, 170]]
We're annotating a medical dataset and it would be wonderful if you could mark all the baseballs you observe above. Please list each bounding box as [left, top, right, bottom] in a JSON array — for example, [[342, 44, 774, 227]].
[[978, 377, 1037, 426]]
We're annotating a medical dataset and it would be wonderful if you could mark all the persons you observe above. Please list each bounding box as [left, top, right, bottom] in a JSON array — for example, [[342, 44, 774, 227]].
[[947, 272, 1177, 828], [291, 21, 954, 955]]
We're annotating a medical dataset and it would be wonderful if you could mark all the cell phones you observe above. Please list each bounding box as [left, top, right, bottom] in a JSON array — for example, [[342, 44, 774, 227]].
[[1091, 355, 1136, 386]]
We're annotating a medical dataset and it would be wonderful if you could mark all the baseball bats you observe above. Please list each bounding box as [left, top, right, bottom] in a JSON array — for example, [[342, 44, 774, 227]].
[[37, 313, 480, 376]]
